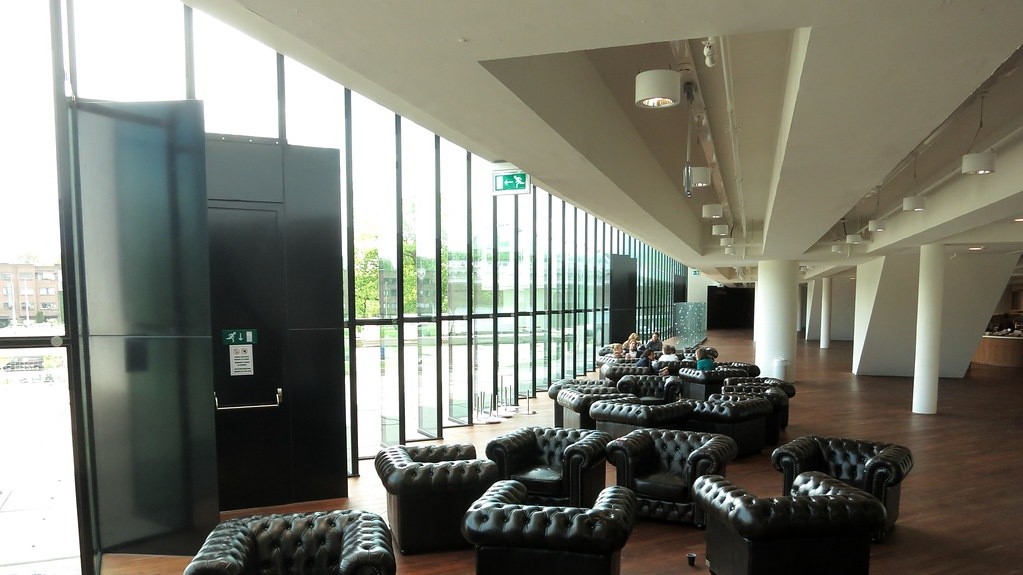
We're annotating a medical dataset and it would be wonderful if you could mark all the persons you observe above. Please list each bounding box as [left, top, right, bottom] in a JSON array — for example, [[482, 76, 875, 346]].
[[620, 339, 646, 358], [997, 313, 1015, 333], [623, 332, 640, 350], [695, 349, 715, 371], [612, 343, 632, 360], [645, 332, 663, 351], [986, 316, 999, 333], [658, 345, 680, 362], [636, 349, 669, 376]]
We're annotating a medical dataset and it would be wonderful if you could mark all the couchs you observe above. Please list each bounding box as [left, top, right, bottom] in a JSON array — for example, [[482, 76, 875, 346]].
[[182, 342, 914, 575]]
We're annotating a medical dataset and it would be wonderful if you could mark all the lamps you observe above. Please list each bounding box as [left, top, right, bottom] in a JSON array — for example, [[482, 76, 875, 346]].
[[902, 154, 927, 212], [831, 235, 861, 253], [683, 167, 710, 187], [869, 186, 886, 232], [634, 70, 680, 109], [712, 225, 736, 255], [961, 96, 995, 175], [702, 204, 722, 218], [703, 42, 719, 69]]
